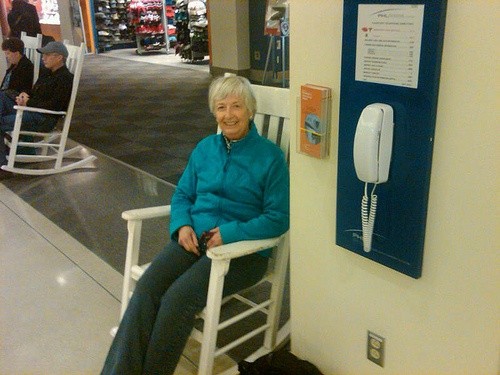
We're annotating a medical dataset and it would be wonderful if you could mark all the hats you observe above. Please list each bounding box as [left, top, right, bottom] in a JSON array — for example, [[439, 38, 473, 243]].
[[36, 41, 69, 58]]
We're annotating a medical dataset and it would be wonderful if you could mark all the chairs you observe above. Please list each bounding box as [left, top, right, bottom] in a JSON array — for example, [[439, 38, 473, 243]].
[[20, 31, 43, 86], [1, 40, 97, 175], [110, 72, 292, 375]]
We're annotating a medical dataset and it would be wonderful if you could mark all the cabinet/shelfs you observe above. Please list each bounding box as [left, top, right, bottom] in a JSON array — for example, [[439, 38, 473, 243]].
[[93, 0, 209, 64]]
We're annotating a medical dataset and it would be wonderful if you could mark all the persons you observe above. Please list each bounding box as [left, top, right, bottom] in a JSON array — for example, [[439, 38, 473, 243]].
[[100, 76, 291, 375], [0, 0, 72, 177]]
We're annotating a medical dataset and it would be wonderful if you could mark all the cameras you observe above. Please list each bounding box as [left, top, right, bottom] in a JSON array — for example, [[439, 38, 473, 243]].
[[198, 232, 214, 253]]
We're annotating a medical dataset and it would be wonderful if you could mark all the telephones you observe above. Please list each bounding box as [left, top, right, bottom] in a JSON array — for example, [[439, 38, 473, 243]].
[[353, 104, 393, 184]]
